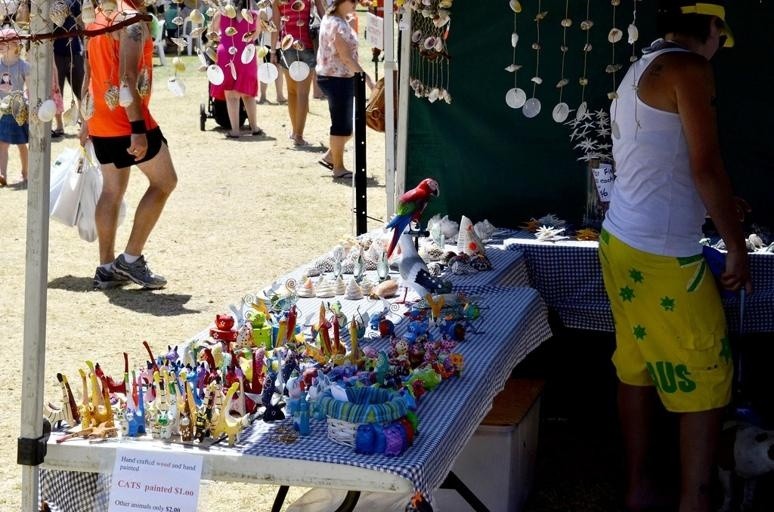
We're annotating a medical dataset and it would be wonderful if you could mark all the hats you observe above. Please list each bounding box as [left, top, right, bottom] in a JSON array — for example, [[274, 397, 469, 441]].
[[680, 0, 736, 49]]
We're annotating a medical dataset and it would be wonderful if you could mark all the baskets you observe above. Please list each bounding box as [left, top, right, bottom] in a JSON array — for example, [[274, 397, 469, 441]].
[[325, 419, 369, 449]]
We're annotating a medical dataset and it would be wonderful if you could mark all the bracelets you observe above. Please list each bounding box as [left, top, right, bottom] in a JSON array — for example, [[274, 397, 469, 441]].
[[128, 119, 147, 135]]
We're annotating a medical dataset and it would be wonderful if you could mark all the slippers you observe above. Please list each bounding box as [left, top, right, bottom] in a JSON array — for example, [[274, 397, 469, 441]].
[[332, 172, 354, 179], [318, 158, 333, 170], [251, 128, 263, 136], [226, 132, 239, 138]]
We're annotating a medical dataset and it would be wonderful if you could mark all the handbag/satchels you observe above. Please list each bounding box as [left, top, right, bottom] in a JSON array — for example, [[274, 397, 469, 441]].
[[309, 2, 321, 55], [367, 78, 384, 132], [49, 140, 125, 241]]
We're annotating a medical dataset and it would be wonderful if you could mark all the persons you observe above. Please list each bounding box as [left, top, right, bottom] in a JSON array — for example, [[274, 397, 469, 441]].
[[76, 1, 177, 290], [210, 0, 374, 178], [596, 2, 754, 511], [0, 0, 90, 187], [145, 0, 193, 40]]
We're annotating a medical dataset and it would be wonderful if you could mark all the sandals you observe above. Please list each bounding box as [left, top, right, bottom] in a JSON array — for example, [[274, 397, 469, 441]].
[[294, 140, 309, 146]]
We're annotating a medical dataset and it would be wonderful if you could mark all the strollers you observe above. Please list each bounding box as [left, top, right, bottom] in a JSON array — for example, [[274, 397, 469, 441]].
[[198, 65, 250, 131]]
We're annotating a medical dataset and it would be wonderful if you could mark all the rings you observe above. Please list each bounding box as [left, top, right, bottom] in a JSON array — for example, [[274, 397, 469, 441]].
[[133, 150, 138, 154]]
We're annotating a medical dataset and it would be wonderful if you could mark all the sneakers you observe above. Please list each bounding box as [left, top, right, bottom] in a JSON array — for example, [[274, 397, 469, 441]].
[[92, 266, 130, 288], [111, 255, 166, 288]]
